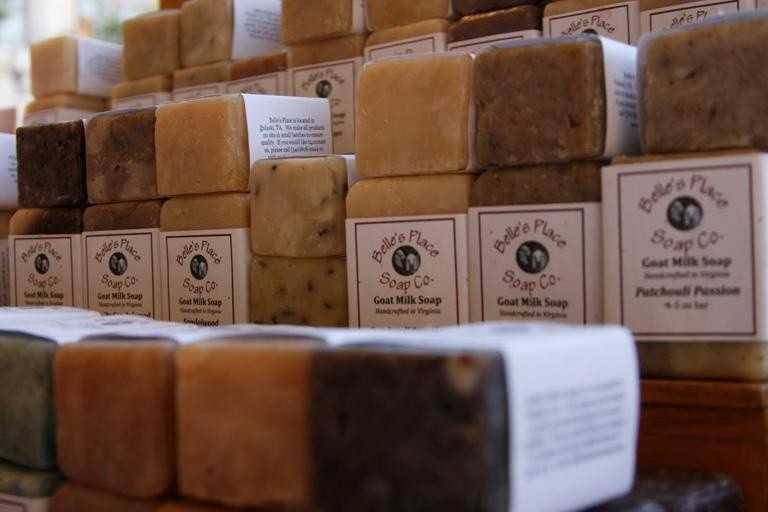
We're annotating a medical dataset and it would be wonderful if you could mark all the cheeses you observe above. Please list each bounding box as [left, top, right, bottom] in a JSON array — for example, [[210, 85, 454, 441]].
[[0, 1, 768, 512]]
